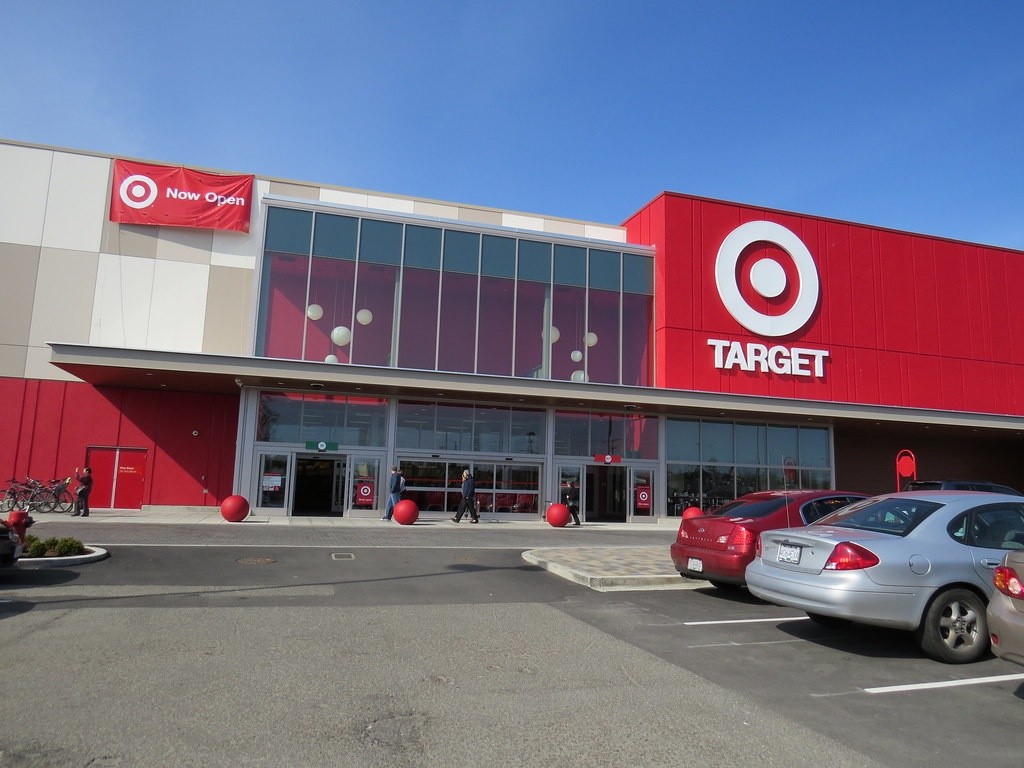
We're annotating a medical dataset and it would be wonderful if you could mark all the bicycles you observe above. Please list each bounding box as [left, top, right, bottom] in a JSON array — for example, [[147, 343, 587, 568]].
[[0, 476, 73, 514]]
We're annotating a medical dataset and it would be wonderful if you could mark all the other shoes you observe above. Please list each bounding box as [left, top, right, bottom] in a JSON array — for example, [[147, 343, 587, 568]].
[[380, 518, 389, 522], [71, 513, 78, 516], [471, 519, 478, 523], [573, 523, 580, 525], [82, 513, 89, 516], [450, 517, 459, 523]]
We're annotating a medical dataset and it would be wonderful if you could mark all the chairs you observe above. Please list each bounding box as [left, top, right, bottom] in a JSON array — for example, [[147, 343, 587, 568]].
[[983, 520, 1024, 549]]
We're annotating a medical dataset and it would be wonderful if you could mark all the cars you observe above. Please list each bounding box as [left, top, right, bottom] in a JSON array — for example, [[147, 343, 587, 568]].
[[985, 551, 1024, 666], [671, 487, 913, 593], [745, 488, 1024, 664]]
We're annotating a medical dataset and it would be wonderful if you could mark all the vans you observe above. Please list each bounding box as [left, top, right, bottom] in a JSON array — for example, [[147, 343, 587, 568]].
[[901, 479, 1024, 541]]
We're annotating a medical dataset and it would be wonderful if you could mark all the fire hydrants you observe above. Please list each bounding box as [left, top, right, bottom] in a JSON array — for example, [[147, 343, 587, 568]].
[[2, 508, 36, 549]]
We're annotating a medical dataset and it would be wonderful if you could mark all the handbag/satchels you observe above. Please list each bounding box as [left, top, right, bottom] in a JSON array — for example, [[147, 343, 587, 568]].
[[400, 488, 407, 499], [74, 487, 84, 495], [566, 500, 575, 508]]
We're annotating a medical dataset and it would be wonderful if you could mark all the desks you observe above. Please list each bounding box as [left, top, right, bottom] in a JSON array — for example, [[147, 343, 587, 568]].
[[702, 497, 729, 509], [668, 496, 699, 513]]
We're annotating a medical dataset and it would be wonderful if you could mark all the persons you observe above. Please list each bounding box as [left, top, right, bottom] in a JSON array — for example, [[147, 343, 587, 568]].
[[71, 467, 93, 518], [449, 469, 479, 524], [380, 466, 406, 522], [564, 480, 581, 526]]
